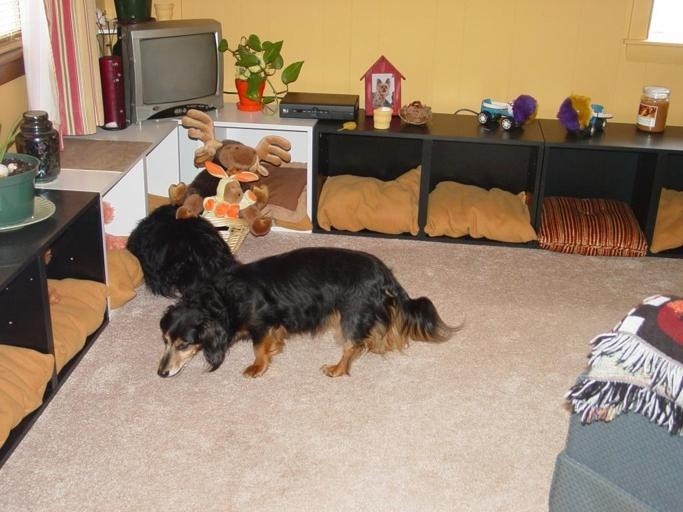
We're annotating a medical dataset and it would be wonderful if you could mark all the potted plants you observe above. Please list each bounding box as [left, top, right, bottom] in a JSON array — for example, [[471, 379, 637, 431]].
[[218, 34, 304, 116], [0, 113, 40, 225]]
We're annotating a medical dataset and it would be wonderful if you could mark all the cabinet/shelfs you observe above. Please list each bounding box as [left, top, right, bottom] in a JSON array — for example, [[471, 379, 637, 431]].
[[163, 103, 318, 233], [312, 114, 545, 249], [0, 188, 109, 468], [535, 119, 683, 258], [35, 121, 179, 285]]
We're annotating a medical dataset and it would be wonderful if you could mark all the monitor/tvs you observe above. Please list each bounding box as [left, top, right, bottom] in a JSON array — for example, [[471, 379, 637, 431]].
[[121, 18, 224, 121]]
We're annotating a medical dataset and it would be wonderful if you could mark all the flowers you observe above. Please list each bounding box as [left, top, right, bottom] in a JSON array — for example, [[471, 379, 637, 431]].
[[96, 9, 118, 56]]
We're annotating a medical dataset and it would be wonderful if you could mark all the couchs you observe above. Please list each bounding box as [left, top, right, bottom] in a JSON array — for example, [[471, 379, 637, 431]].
[[549, 294, 683, 512]]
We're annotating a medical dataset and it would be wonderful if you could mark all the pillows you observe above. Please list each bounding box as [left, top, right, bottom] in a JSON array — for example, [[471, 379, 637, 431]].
[[650, 187, 683, 254], [147, 193, 170, 215], [107, 236, 145, 310], [0, 345, 55, 447], [245, 162, 313, 231], [538, 197, 649, 256], [424, 181, 539, 242], [317, 165, 421, 236], [47, 278, 112, 374]]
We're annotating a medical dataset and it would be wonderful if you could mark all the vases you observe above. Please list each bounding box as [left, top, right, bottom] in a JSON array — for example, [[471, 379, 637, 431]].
[[99, 56, 126, 129]]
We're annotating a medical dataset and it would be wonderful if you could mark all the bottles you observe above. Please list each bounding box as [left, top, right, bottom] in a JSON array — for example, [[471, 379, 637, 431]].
[[16, 111, 61, 182], [635, 86, 670, 133]]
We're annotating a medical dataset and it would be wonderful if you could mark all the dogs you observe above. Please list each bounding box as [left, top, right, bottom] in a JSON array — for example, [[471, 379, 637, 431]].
[[158, 246, 468, 379], [372, 78, 392, 107], [125, 203, 237, 300]]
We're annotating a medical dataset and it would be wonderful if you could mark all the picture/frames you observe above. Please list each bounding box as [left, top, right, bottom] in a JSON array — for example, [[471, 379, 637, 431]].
[[360, 54, 406, 116]]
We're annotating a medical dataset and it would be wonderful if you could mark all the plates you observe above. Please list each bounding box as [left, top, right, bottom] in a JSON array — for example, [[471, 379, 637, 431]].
[[0, 196, 56, 233]]
